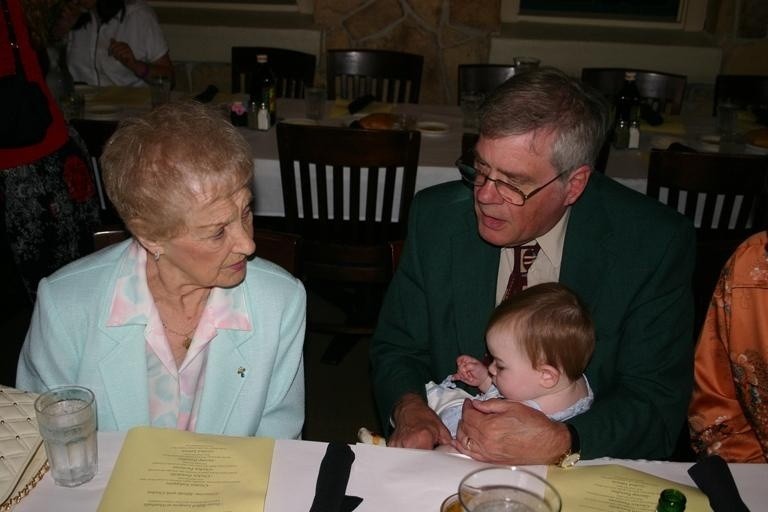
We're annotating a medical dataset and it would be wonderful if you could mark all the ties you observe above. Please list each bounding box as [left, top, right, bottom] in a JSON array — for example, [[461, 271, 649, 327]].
[[502, 242, 541, 301]]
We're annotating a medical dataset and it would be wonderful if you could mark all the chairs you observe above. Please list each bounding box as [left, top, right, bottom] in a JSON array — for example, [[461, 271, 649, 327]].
[[326, 47, 423, 105], [458, 61, 533, 107], [457, 132, 615, 191], [266, 120, 424, 364], [711, 69, 767, 126], [229, 48, 318, 99], [639, 143, 768, 310], [582, 66, 686, 115]]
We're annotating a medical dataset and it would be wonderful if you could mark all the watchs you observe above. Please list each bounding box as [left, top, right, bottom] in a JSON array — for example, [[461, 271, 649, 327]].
[[552, 421, 584, 468]]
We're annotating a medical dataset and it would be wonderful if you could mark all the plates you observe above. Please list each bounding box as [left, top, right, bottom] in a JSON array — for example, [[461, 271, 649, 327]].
[[414, 123, 450, 137], [743, 128, 767, 151], [74, 84, 99, 98], [89, 102, 121, 116]]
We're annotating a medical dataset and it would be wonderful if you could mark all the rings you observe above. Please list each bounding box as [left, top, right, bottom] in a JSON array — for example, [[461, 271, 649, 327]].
[[464, 437, 472, 450]]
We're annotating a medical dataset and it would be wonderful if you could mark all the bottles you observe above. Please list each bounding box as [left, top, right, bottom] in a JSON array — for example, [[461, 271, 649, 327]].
[[655, 486, 687, 511], [250, 54, 275, 128], [246, 102, 271, 131], [613, 121, 640, 150], [616, 71, 641, 127]]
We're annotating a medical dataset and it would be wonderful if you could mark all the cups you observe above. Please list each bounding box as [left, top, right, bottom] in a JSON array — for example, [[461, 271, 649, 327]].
[[440, 492, 459, 512], [512, 55, 542, 75], [303, 84, 327, 120], [33, 386, 100, 489], [459, 91, 485, 131], [715, 102, 736, 143], [457, 463, 564, 511], [148, 71, 171, 108]]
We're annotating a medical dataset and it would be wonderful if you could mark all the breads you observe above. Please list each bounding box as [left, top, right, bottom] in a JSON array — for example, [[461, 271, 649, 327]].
[[747, 128, 768, 149], [359, 110, 404, 130]]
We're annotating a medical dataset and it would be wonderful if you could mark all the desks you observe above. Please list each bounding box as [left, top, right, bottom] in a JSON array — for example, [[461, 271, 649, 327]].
[[13, 422, 768, 509], [64, 80, 768, 238]]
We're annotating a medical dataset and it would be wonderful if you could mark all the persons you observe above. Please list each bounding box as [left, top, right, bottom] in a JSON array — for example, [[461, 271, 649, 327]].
[[370, 67, 701, 460], [358, 282, 596, 448], [43, 0, 174, 87], [689, 230, 766, 464], [1, 0, 103, 309], [15, 97, 306, 442]]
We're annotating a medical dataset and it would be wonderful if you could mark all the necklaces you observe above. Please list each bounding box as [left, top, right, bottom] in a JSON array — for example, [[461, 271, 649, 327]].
[[163, 316, 197, 349]]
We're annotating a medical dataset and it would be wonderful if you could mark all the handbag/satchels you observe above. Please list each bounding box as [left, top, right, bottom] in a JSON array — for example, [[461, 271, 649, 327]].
[[0, 74, 52, 149]]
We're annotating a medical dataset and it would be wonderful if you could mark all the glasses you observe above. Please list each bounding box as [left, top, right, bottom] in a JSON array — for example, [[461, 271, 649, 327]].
[[456, 142, 570, 206]]
